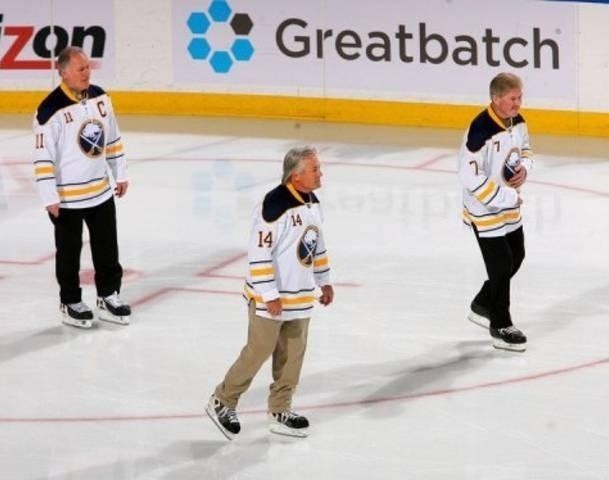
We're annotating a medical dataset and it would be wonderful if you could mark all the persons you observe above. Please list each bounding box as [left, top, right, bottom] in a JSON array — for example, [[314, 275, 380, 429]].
[[30, 44, 133, 321], [453, 72, 533, 345], [211, 144, 334, 434]]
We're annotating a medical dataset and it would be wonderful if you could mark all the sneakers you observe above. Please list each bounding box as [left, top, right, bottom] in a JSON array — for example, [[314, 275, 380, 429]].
[[267, 408, 311, 430], [59, 300, 94, 320], [208, 393, 241, 434], [470, 296, 492, 320], [489, 323, 527, 344], [96, 289, 132, 317]]
